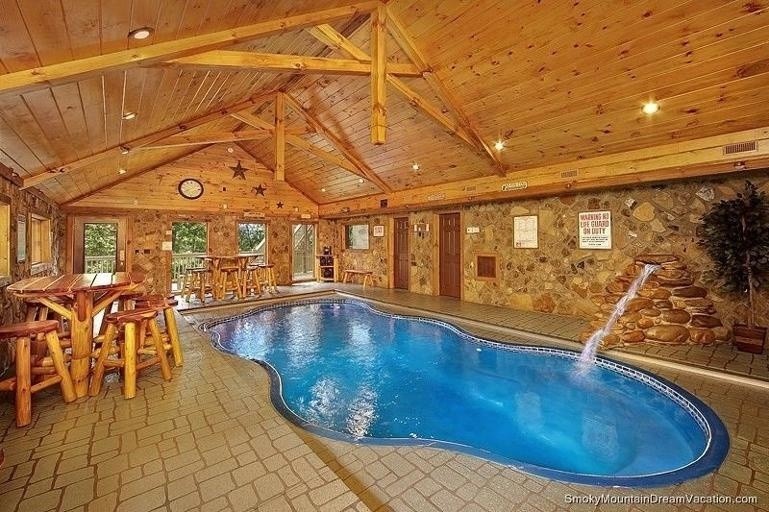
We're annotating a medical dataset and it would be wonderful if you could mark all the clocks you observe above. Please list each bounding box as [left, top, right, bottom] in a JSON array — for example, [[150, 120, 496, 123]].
[[178, 178, 203, 199]]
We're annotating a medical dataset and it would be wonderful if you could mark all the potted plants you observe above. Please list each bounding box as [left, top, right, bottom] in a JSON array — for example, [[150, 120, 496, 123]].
[[695, 182, 769, 354]]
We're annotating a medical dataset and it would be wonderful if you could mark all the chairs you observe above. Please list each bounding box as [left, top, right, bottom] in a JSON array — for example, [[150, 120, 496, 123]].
[[180, 250, 279, 302], [0, 271, 183, 428]]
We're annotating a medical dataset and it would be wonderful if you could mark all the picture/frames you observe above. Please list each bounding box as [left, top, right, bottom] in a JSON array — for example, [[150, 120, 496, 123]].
[[512, 214, 539, 249], [577, 211, 613, 251]]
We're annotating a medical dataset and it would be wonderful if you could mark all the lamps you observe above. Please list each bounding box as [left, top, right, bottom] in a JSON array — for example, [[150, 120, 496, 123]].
[[128, 28, 155, 41], [122, 111, 138, 121], [120, 145, 130, 155]]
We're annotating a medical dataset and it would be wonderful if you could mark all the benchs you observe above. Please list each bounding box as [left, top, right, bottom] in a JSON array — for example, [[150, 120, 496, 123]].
[[341, 270, 375, 288]]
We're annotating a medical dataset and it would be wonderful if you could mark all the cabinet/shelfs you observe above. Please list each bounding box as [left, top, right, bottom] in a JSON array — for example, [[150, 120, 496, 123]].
[[316, 254, 338, 283]]
